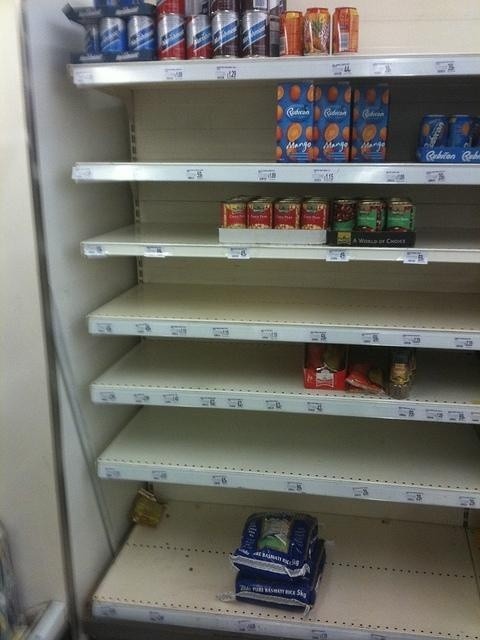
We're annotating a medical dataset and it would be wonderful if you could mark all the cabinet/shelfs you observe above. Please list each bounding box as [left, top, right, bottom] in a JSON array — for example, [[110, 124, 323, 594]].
[[67, 51, 480, 640]]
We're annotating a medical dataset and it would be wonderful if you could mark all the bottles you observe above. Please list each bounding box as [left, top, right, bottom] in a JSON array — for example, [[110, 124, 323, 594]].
[[266, 17, 281, 56], [81, 0, 267, 62], [220, 193, 331, 229], [332, 6, 359, 54], [269, 0, 287, 20], [279, 10, 303, 56], [333, 195, 416, 233], [303, 7, 331, 55], [416, 111, 480, 147]]
